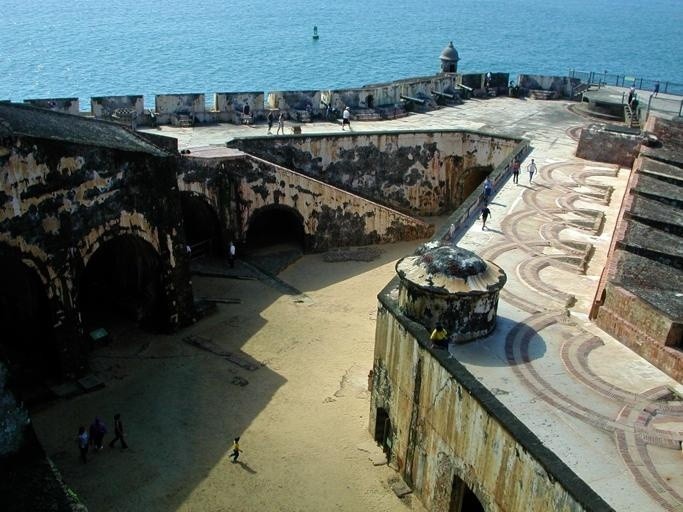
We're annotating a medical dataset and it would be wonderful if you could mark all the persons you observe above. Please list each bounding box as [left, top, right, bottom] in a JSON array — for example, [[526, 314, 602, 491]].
[[481, 203, 491, 230], [228, 241, 236, 269], [430, 326, 448, 351], [89, 417, 107, 451], [483, 176, 492, 203], [227, 436, 243, 463], [510, 80, 519, 98], [512, 161, 521, 186], [527, 159, 537, 183], [651, 81, 660, 98], [244, 102, 352, 135], [77, 427, 89, 464], [108, 414, 128, 449], [628, 84, 639, 118]]
